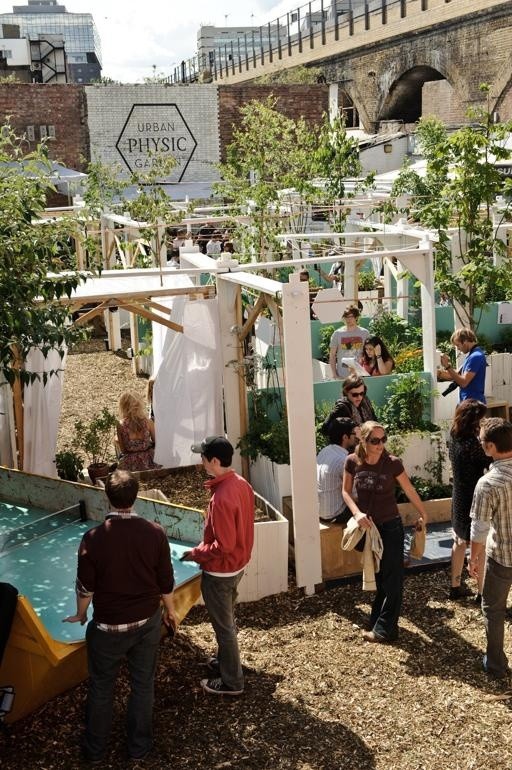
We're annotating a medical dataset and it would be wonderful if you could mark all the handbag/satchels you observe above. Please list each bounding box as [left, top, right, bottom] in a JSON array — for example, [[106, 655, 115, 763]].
[[409, 519, 426, 561]]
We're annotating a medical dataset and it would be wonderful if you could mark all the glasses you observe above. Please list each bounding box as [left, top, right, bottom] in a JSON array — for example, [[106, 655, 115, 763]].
[[370, 435, 387, 445], [477, 436, 485, 442], [352, 391, 365, 397]]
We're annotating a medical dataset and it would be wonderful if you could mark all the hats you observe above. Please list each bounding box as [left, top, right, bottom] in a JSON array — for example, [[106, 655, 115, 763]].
[[191, 436, 234, 459]]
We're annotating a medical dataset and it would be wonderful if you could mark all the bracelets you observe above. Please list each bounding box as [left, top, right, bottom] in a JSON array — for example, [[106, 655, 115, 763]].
[[444, 365, 451, 370], [437, 371, 442, 377]]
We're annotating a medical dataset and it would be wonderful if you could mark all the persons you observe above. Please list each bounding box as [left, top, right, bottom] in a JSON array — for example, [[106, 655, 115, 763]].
[[341, 421, 430, 643], [316, 417, 360, 525], [468, 415, 512, 679], [62, 467, 178, 765], [448, 399, 494, 605], [438, 327, 490, 405], [113, 390, 163, 472], [321, 373, 378, 455], [180, 435, 256, 696]]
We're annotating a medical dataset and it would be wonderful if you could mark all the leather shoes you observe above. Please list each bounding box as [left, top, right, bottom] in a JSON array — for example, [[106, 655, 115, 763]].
[[451, 581, 465, 601], [362, 631, 388, 643], [362, 615, 373, 628]]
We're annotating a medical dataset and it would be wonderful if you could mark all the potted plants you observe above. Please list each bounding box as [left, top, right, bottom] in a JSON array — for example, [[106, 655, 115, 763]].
[[67, 406, 123, 485]]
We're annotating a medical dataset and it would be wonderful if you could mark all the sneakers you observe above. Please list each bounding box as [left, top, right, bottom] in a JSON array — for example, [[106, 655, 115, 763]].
[[482, 654, 505, 679], [207, 657, 220, 673], [200, 677, 244, 696]]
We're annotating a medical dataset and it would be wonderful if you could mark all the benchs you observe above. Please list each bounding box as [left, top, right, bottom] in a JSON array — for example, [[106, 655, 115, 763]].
[[282, 495, 367, 578]]
